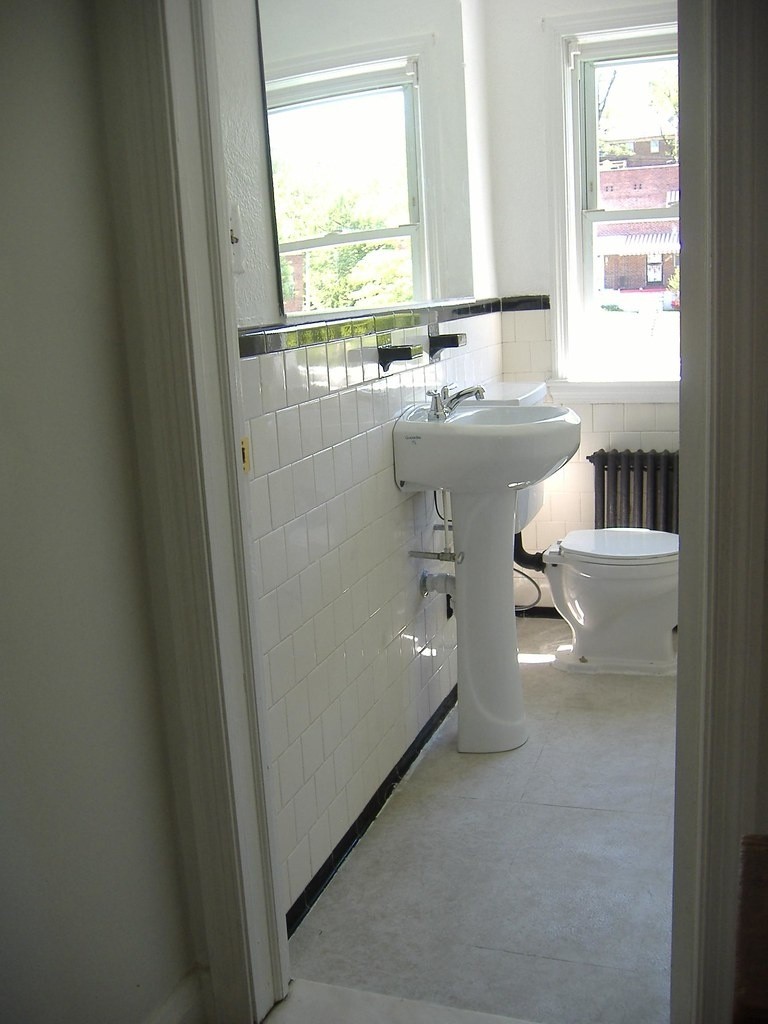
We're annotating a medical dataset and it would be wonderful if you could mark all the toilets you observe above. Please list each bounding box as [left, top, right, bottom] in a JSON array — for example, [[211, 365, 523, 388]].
[[542, 527, 679, 670]]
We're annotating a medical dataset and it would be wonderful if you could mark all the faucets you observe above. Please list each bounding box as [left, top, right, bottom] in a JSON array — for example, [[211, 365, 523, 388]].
[[441, 385, 486, 415]]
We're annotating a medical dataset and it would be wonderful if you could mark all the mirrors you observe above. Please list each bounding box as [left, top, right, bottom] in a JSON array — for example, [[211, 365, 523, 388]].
[[255, 0, 455, 319]]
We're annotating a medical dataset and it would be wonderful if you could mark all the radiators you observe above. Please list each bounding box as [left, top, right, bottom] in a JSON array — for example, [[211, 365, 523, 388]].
[[585, 449, 680, 533]]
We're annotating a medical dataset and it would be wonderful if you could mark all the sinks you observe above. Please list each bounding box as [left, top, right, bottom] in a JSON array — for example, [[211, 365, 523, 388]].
[[440, 404, 582, 494]]
